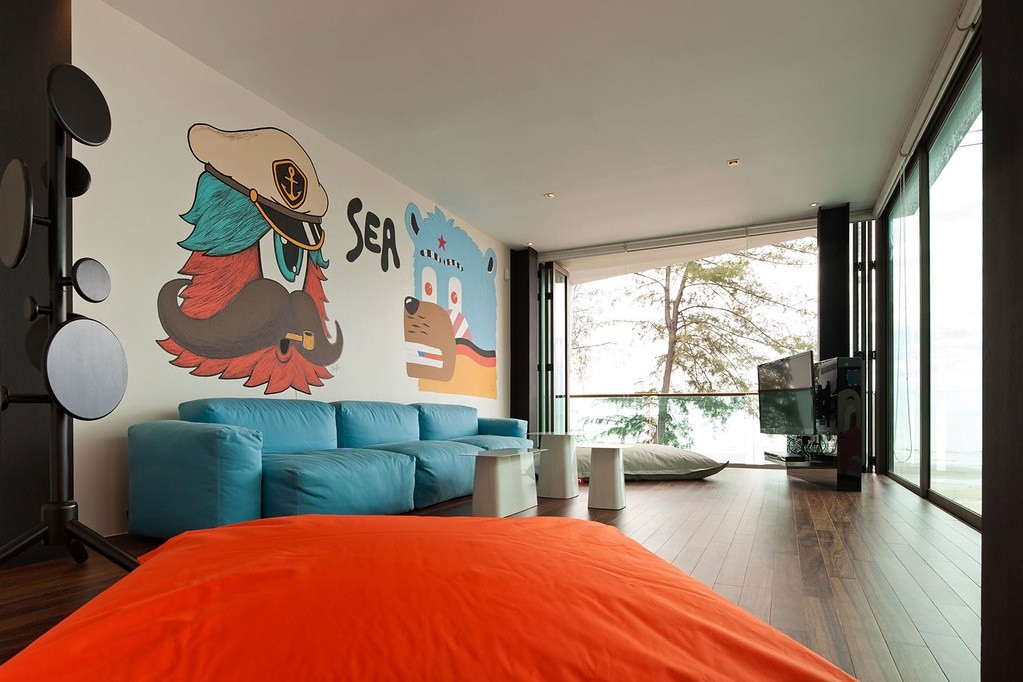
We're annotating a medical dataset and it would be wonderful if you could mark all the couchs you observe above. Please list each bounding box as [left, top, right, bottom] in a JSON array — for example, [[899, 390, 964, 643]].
[[128, 399, 530, 537]]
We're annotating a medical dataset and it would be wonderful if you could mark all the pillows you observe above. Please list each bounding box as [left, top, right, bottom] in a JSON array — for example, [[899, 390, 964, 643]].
[[532, 443, 730, 480]]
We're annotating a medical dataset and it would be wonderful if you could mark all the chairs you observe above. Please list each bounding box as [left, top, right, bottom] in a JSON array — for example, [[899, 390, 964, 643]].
[[0, 514, 869, 682]]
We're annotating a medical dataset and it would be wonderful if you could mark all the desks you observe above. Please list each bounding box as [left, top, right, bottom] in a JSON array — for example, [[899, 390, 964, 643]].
[[460, 448, 549, 517], [576, 443, 636, 510], [523, 432, 587, 499]]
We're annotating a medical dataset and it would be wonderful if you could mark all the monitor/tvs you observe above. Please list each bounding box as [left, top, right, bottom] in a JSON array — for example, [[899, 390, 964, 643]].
[[757, 351, 817, 436]]
